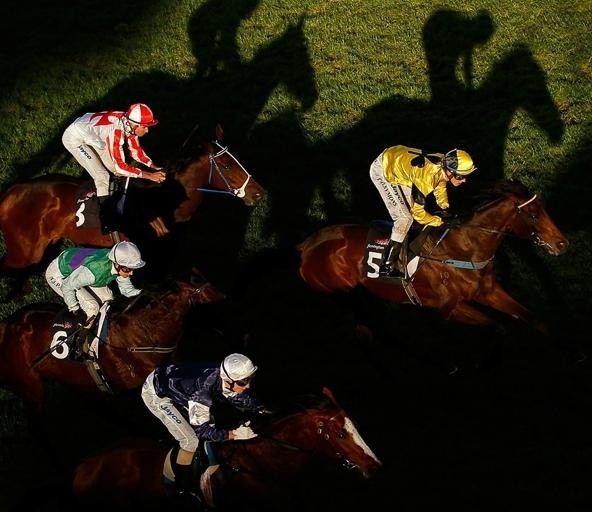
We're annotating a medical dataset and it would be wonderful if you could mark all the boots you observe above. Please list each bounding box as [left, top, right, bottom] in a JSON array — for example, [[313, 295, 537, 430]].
[[98, 195, 116, 235], [68, 325, 90, 362], [379, 239, 405, 277]]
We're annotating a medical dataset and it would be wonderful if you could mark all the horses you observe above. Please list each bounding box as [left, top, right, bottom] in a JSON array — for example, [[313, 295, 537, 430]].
[[296, 174, 568, 327], [1, 267, 217, 398], [0, 123, 265, 269], [73, 387, 382, 512]]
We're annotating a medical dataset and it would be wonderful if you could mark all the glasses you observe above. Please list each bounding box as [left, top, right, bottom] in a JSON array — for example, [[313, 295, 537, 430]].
[[114, 241, 131, 273], [223, 358, 248, 386], [444, 154, 464, 180]]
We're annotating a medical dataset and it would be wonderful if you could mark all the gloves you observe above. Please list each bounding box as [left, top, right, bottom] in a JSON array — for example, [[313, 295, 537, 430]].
[[232, 421, 258, 440], [434, 209, 461, 228], [75, 308, 87, 324]]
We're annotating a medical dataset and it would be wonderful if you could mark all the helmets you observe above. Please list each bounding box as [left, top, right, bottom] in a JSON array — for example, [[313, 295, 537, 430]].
[[122, 103, 159, 127], [108, 241, 146, 269], [220, 353, 258, 383], [440, 149, 478, 175]]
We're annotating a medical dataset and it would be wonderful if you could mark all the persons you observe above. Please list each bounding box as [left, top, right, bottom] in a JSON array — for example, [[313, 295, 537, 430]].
[[44, 238, 147, 365], [367, 145, 478, 280], [139, 353, 275, 497], [60, 102, 167, 233]]
